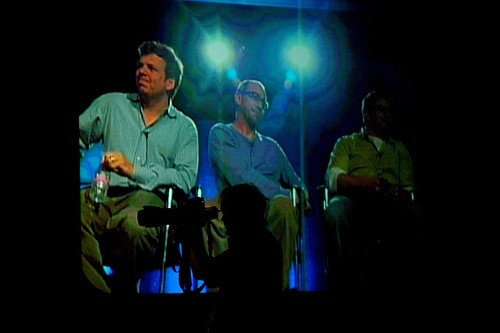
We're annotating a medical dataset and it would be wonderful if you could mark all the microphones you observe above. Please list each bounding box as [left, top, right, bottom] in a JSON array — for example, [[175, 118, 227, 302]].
[[378, 153, 383, 159], [145, 132, 148, 140]]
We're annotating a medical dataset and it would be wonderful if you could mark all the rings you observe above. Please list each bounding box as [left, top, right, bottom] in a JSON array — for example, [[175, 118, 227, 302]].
[[111, 157, 114, 162]]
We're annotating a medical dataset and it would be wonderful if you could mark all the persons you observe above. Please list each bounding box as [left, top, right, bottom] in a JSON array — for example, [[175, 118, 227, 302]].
[[79, 41, 199, 295], [324, 89, 473, 281], [207, 79, 311, 293]]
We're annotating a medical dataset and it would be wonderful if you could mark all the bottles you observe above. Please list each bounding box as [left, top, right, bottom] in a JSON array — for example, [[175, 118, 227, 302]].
[[90, 165, 110, 202]]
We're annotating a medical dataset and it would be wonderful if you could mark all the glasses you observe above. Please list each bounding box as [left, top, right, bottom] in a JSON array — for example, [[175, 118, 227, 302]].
[[244, 90, 269, 109]]
